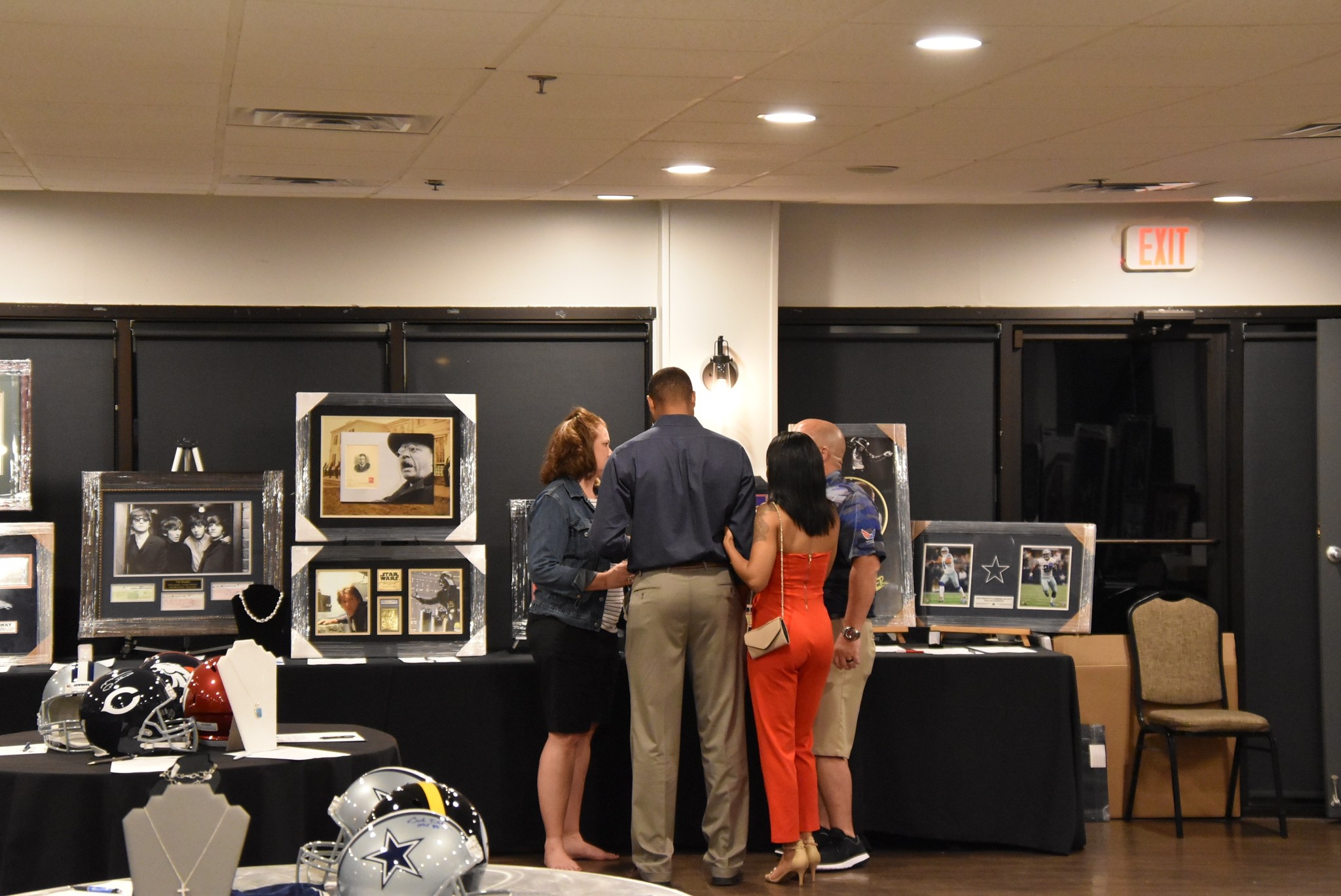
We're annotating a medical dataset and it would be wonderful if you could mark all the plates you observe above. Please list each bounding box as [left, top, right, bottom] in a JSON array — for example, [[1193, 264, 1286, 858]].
[[985, 639, 1023, 644]]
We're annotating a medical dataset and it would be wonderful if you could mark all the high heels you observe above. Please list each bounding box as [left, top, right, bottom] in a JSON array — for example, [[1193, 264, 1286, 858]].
[[765, 837, 821, 886]]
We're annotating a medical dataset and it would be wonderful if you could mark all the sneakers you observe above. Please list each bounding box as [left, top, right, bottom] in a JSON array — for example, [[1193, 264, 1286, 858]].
[[775, 823, 870, 870]]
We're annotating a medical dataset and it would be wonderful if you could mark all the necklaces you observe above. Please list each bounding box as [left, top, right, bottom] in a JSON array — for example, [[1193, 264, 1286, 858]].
[[239, 590, 284, 623], [144, 802, 231, 896]]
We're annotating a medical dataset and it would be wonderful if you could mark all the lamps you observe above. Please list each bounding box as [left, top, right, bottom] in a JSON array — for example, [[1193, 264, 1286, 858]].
[[702, 336, 739, 394]]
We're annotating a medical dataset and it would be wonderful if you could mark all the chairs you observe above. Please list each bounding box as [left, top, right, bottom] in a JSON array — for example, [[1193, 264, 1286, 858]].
[[1125, 592, 1292, 841]]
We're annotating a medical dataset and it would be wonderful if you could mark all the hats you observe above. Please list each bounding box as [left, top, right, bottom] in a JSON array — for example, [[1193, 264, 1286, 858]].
[[388, 432, 434, 459]]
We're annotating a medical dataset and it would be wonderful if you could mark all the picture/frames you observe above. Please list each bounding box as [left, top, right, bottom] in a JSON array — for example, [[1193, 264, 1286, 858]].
[[295, 390, 476, 541], [911, 520, 1097, 634], [786, 422, 916, 627], [78, 469, 282, 639], [291, 544, 487, 659], [0, 522, 55, 667], [0, 359, 34, 512]]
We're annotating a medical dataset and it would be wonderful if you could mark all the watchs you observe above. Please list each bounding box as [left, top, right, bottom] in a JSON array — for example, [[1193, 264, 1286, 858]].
[[842, 626, 861, 643]]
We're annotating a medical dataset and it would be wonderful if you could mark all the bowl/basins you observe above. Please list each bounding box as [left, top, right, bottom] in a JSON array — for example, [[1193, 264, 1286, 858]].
[[944, 632, 979, 643]]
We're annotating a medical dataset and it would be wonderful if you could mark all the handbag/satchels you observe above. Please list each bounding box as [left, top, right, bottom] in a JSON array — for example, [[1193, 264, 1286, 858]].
[[744, 616, 790, 659]]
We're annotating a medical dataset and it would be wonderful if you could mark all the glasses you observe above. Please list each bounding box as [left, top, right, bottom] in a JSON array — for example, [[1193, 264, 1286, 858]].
[[398, 445, 417, 454], [132, 516, 150, 521]]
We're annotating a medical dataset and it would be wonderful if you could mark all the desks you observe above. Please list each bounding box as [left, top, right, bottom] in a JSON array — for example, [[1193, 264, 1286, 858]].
[[22, 864, 687, 895], [0, 731, 403, 893], [0, 654, 1088, 860]]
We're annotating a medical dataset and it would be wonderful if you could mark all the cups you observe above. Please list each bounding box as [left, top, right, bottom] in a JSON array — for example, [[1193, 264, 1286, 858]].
[[996, 634, 1015, 641]]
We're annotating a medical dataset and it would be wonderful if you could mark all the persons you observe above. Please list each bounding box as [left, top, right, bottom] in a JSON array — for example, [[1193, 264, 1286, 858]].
[[795, 418, 890, 873], [411, 571, 460, 632], [526, 403, 633, 874], [590, 365, 756, 887], [372, 433, 434, 504], [126, 507, 166, 573], [184, 513, 233, 573], [929, 546, 967, 603], [197, 515, 234, 572], [1028, 548, 1059, 607], [354, 454, 370, 473], [153, 516, 196, 575], [317, 586, 368, 633], [728, 430, 840, 887]]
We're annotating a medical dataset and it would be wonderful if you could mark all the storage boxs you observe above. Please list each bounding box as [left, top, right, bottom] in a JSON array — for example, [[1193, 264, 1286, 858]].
[[1051, 633, 1242, 818]]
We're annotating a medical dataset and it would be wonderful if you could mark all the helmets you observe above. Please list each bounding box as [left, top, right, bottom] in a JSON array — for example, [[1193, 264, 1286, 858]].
[[183, 653, 241, 750], [37, 663, 114, 753], [79, 668, 199, 756], [140, 651, 204, 720], [296, 768, 512, 896]]
